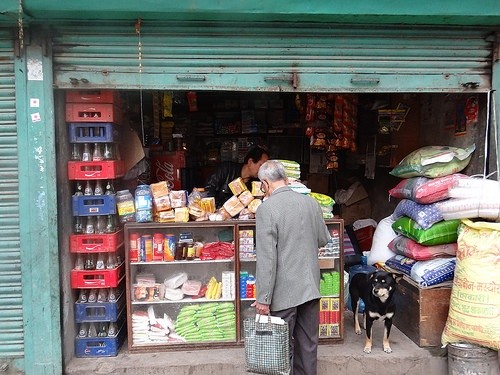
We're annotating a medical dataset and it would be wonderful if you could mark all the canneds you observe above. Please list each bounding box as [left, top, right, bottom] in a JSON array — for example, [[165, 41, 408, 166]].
[[174, 241, 196, 261]]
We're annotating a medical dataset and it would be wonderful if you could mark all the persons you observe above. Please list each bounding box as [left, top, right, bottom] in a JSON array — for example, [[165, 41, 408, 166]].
[[204, 149, 270, 209], [250, 160, 330, 375]]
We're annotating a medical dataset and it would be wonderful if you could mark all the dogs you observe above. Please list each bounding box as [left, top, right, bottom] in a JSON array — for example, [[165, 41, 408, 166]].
[[349, 269, 405, 354]]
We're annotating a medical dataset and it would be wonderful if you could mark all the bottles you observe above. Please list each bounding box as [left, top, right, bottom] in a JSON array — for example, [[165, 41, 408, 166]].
[[75, 216, 116, 243], [71, 141, 114, 172], [116, 185, 153, 224], [129, 232, 203, 260], [75, 252, 122, 278], [75, 179, 114, 204], [78, 287, 122, 314], [79, 322, 119, 347]]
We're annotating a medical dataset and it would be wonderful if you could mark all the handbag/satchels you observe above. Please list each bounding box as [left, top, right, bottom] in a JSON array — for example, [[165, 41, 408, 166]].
[[242, 313, 292, 375]]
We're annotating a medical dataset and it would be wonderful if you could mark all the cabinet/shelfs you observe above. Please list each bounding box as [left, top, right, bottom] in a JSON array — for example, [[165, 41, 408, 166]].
[[359, 93, 425, 168], [124, 215, 346, 345], [154, 92, 305, 195]]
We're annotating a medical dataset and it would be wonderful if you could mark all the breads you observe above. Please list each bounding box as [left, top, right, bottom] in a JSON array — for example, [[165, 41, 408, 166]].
[[150, 177, 266, 224]]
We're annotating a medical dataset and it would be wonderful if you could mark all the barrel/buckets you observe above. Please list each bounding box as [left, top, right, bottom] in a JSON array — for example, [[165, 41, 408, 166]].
[[448, 341, 500, 375]]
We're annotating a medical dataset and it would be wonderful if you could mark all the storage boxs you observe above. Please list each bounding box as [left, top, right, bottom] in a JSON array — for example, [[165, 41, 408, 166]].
[[70, 257, 125, 289], [337, 183, 371, 226], [74, 291, 127, 323], [375, 263, 452, 348], [75, 325, 125, 358], [71, 195, 118, 217], [65, 88, 124, 143], [69, 229, 124, 254], [68, 159, 125, 180]]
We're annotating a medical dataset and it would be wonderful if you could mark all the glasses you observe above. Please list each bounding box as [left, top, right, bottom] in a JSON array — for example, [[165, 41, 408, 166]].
[[260, 180, 269, 193]]
